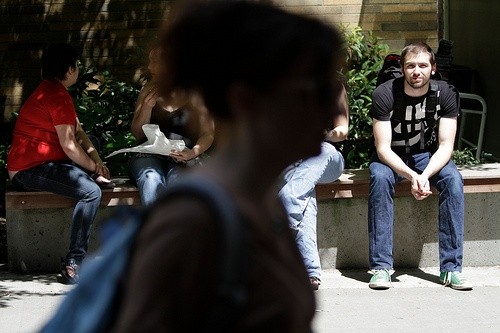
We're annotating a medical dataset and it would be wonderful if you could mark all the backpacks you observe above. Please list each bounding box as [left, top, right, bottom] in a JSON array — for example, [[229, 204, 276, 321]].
[[376, 54, 461, 154]]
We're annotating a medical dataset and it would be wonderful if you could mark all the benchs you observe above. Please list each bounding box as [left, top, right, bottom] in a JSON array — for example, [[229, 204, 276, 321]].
[[6, 169, 500, 273]]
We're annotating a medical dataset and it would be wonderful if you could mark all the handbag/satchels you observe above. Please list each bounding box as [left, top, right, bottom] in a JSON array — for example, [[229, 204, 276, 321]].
[[35, 174, 239, 333]]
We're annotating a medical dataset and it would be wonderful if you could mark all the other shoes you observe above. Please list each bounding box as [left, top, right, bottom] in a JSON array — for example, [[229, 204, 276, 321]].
[[310, 277, 321, 291]]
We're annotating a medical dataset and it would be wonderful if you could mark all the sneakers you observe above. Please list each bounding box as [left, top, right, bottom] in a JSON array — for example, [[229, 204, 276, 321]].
[[91, 173, 115, 189], [439, 271, 473, 290], [369, 268, 392, 288], [57, 265, 80, 284]]
